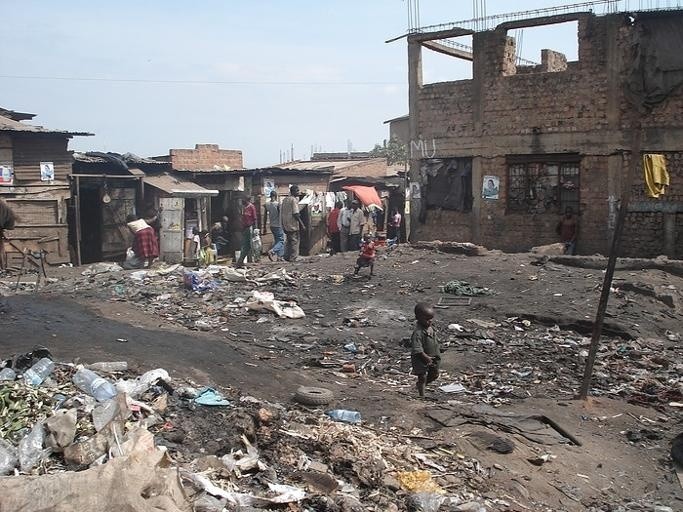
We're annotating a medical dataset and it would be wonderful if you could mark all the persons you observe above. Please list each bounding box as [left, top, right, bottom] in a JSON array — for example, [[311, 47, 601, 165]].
[[281, 186, 306, 261], [263, 190, 285, 262], [410, 302, 448, 396], [325, 196, 402, 277], [312, 204, 323, 214], [126, 214, 160, 268], [556, 206, 576, 243], [235, 195, 258, 263], [484, 179, 498, 198], [190, 215, 230, 267], [43, 164, 53, 179], [252, 228, 263, 262]]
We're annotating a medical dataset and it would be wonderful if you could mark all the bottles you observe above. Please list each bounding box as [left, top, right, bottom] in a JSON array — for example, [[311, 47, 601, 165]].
[[87, 361, 127, 372], [71, 368, 116, 403], [0, 366, 16, 380], [325, 408, 360, 422], [22, 357, 53, 387]]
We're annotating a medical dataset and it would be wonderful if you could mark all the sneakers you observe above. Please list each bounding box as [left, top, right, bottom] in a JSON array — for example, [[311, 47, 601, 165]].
[[267, 250, 274, 261], [276, 256, 284, 261]]
